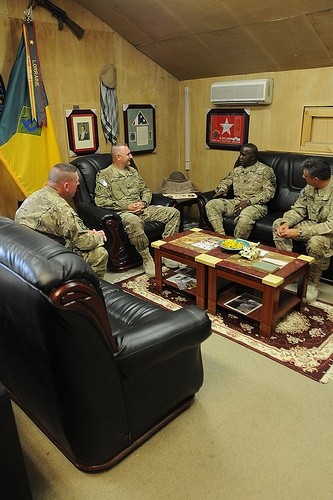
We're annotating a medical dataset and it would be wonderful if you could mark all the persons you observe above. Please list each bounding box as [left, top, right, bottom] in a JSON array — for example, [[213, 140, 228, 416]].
[[15, 163, 108, 279], [205, 143, 276, 240], [94, 144, 180, 277], [272, 157, 333, 304]]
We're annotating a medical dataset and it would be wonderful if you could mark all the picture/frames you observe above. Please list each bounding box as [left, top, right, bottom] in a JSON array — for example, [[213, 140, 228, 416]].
[[65, 109, 100, 157], [122, 103, 157, 154], [204, 106, 250, 151]]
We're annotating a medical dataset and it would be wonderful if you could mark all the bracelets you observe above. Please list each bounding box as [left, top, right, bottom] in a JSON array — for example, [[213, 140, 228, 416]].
[[247, 200, 251, 206], [297, 231, 302, 240]]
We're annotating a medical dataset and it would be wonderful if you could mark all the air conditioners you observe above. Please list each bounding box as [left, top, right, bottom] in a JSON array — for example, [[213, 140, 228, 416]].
[[209, 77, 275, 105]]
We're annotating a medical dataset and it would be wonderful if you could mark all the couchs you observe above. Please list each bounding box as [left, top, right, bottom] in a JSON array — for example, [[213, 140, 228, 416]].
[[67, 153, 180, 273], [208, 150, 333, 286], [0, 215, 213, 473]]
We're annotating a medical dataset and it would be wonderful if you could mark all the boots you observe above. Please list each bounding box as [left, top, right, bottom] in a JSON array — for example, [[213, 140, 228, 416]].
[[162, 257, 179, 268], [140, 247, 156, 277]]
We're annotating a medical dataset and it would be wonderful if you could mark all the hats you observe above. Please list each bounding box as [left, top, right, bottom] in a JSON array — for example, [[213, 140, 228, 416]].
[[100, 64, 117, 88]]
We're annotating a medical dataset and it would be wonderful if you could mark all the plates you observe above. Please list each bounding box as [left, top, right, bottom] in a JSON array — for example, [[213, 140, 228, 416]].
[[217, 239, 250, 251]]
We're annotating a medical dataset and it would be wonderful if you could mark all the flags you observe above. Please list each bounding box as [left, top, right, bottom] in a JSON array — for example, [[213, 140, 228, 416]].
[[0, 21, 62, 197]]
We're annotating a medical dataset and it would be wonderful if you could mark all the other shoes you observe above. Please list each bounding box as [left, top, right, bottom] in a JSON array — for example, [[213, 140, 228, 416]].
[[306, 283, 320, 303]]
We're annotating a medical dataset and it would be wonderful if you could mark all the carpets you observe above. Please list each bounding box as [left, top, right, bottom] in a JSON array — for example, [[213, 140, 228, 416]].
[[112, 261, 333, 385]]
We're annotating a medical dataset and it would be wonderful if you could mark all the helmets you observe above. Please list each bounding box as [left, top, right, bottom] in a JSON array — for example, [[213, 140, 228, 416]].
[[167, 172, 187, 182]]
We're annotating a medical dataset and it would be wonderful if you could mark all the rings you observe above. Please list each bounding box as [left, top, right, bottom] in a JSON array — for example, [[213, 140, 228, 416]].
[[239, 207, 242, 209]]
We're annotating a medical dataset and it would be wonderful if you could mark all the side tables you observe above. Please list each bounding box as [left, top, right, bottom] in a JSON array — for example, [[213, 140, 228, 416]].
[[176, 192, 202, 233]]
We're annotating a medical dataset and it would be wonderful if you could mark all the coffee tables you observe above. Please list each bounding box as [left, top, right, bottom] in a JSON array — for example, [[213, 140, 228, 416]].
[[150, 228, 316, 338]]
